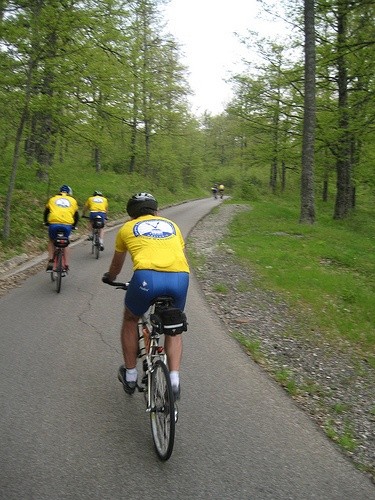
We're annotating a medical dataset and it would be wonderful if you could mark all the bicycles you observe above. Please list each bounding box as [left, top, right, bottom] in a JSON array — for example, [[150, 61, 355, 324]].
[[42, 222, 79, 294], [212, 189, 225, 199], [81, 215, 109, 259], [103, 278, 189, 462]]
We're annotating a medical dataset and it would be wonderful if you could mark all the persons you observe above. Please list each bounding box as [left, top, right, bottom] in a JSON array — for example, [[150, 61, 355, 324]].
[[43, 185, 79, 275], [81, 192, 109, 251], [212, 183, 224, 198], [104, 194, 190, 404]]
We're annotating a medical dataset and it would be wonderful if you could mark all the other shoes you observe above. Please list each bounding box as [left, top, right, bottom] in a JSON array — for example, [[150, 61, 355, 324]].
[[46, 265, 52, 270], [64, 265, 70, 272], [87, 236, 93, 241], [100, 244, 104, 251]]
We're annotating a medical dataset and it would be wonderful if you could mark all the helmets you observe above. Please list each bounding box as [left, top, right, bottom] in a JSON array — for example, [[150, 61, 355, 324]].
[[127, 193, 158, 216], [94, 191, 103, 196], [61, 185, 72, 194]]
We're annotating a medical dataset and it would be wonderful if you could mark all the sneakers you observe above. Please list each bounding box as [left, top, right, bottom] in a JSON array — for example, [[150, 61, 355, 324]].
[[118, 364, 136, 394], [164, 385, 180, 401]]
